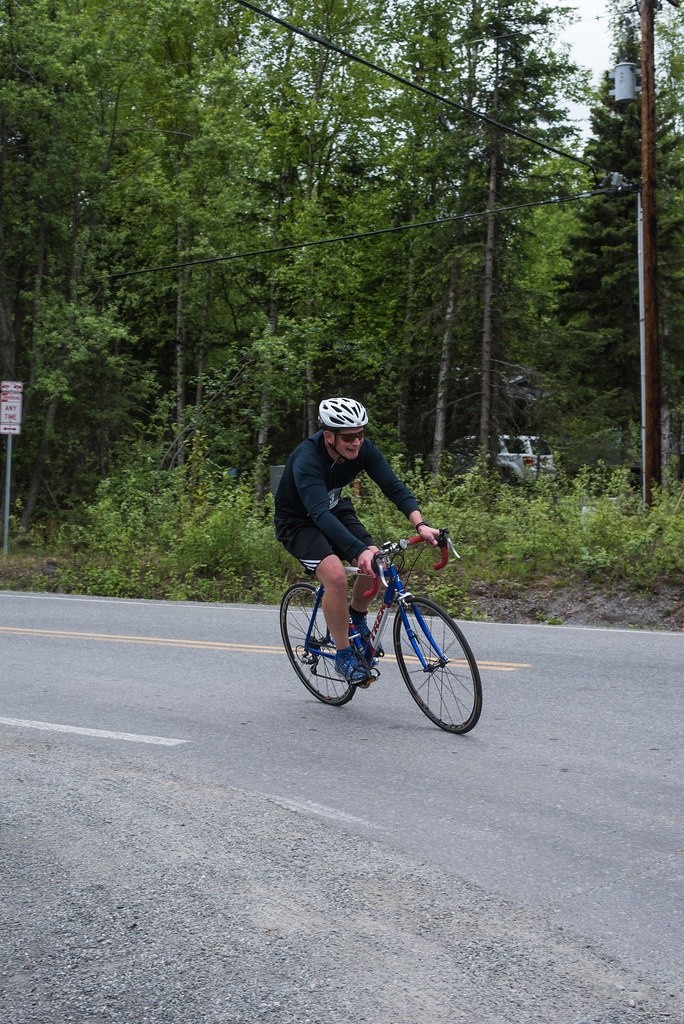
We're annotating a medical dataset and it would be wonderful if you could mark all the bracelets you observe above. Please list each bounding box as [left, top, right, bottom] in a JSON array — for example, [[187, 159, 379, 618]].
[[416, 521, 430, 533]]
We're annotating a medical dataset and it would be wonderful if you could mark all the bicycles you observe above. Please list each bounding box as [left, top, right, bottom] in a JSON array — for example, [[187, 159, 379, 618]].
[[278, 526, 484, 734]]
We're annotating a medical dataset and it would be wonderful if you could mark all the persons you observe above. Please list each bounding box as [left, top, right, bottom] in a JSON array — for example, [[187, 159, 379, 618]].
[[274, 396, 452, 685]]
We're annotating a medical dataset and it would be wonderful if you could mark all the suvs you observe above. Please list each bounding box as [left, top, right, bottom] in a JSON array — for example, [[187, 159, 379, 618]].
[[445, 435, 556, 486]]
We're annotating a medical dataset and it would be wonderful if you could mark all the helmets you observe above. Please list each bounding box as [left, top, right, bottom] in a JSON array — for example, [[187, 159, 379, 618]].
[[318, 397, 368, 431]]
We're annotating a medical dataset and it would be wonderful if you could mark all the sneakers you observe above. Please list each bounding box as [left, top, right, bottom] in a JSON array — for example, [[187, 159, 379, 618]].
[[334, 647, 371, 684], [346, 614, 382, 655]]
